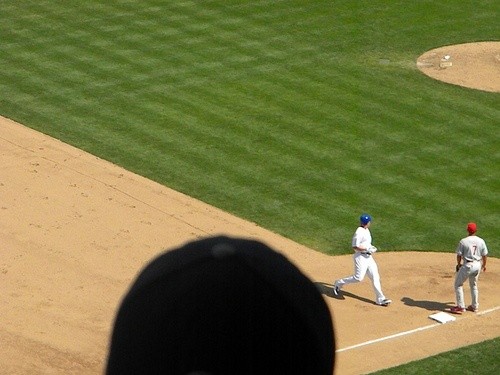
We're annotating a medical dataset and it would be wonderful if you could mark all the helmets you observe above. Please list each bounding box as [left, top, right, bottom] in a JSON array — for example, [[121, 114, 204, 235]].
[[360, 215, 371, 225]]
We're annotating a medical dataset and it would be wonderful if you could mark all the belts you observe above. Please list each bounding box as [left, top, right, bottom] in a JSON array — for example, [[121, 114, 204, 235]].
[[361, 252, 371, 255], [466, 260, 472, 262]]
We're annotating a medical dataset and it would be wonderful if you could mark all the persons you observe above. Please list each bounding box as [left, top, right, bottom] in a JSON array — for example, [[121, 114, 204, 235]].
[[334, 214, 392, 305], [104, 234, 336, 375], [450, 223, 488, 313]]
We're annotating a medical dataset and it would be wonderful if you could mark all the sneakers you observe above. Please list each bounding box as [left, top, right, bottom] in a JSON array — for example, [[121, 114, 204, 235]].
[[450, 306, 465, 313], [334, 280, 340, 295], [376, 299, 392, 305], [468, 305, 478, 311]]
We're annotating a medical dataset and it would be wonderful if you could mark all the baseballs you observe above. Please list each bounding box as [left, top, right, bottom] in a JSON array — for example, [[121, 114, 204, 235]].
[[443, 56, 450, 59]]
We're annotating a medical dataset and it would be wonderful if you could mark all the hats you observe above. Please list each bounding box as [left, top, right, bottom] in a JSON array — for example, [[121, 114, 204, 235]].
[[468, 223, 477, 233]]
